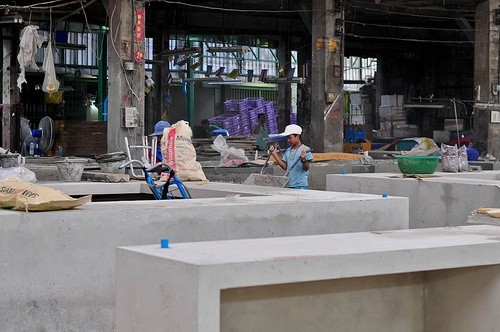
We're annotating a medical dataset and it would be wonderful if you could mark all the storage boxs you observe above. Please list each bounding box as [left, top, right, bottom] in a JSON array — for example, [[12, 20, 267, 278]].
[[207, 92, 477, 144]]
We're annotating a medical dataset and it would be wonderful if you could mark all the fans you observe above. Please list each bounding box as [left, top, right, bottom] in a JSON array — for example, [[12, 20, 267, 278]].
[[30, 115, 56, 158]]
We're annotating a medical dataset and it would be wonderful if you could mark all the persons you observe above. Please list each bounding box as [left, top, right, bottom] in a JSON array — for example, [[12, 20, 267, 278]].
[[253, 113, 268, 150], [148, 120, 172, 164], [270, 125, 313, 189], [200, 119, 222, 131]]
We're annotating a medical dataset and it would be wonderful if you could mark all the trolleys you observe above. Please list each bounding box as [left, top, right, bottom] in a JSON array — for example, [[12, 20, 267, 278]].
[[117, 159, 190, 199], [241, 142, 314, 186]]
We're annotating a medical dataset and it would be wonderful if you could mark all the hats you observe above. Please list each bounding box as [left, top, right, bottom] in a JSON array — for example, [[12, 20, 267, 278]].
[[148, 121, 170, 136], [279, 124, 302, 136]]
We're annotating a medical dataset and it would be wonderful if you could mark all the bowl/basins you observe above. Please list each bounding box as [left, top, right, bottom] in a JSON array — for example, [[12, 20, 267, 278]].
[[395, 156, 441, 174]]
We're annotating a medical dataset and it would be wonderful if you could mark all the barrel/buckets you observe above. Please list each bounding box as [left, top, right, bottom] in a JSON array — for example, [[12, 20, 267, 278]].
[[55, 159, 88, 181], [96, 152, 127, 174]]
